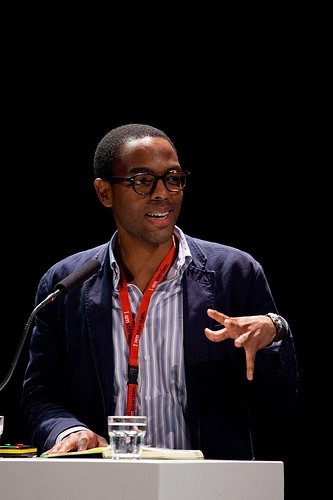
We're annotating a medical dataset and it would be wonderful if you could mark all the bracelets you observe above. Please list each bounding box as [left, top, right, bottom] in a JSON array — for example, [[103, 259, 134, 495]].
[[267, 313, 287, 339]]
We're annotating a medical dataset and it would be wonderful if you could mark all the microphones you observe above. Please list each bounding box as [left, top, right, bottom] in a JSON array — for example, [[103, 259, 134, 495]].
[[0, 259, 101, 391]]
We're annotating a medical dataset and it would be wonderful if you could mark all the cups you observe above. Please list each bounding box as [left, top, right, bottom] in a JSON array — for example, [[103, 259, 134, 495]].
[[0, 415, 4, 447], [108, 416, 147, 460]]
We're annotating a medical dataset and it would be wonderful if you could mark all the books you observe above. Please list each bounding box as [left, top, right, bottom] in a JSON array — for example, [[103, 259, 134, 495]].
[[43, 446, 206, 460]]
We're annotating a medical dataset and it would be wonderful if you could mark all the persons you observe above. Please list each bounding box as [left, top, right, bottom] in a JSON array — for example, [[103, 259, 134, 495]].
[[20, 124, 295, 461]]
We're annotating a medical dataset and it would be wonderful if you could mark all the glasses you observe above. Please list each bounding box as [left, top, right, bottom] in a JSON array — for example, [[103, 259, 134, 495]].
[[109, 170, 187, 197]]
